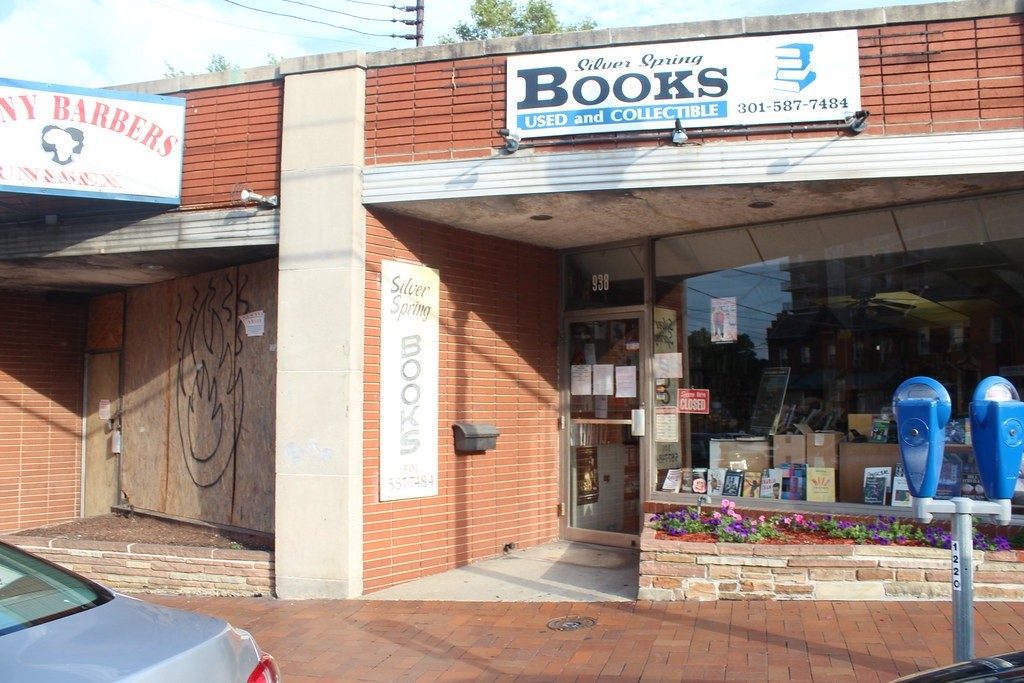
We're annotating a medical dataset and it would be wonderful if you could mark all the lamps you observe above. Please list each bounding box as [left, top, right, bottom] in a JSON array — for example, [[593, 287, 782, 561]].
[[672, 118, 690, 143], [44, 214, 62, 226], [240, 190, 279, 207], [496, 127, 522, 153], [845, 106, 872, 134]]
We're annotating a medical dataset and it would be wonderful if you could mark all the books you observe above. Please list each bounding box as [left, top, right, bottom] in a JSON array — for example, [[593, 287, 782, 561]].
[[662, 453, 988, 507]]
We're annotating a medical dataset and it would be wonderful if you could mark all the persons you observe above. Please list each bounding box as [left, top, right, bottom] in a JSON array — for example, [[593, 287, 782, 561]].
[[746, 478, 761, 497], [773, 484, 780, 499], [797, 400, 826, 432]]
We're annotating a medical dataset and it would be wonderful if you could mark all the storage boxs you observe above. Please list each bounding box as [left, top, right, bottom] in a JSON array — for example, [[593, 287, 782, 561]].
[[806, 431, 846, 469], [772, 423, 814, 465]]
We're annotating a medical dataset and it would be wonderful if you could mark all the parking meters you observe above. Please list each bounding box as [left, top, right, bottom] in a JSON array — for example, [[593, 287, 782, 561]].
[[887, 375, 1024, 665]]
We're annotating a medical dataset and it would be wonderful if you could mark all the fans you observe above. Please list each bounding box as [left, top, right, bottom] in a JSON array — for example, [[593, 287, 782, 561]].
[[815, 287, 916, 317]]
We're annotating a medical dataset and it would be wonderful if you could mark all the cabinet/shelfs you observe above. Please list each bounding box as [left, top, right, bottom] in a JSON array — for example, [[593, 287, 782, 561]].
[[839, 440, 979, 501], [709, 439, 771, 471], [847, 413, 891, 440]]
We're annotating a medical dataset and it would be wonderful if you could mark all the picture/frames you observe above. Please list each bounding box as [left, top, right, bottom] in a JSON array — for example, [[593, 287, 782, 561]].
[[750, 366, 791, 435]]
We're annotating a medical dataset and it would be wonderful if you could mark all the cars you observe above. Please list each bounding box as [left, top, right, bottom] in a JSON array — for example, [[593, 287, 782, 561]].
[[0, 538, 283, 683], [691, 431, 753, 459]]
